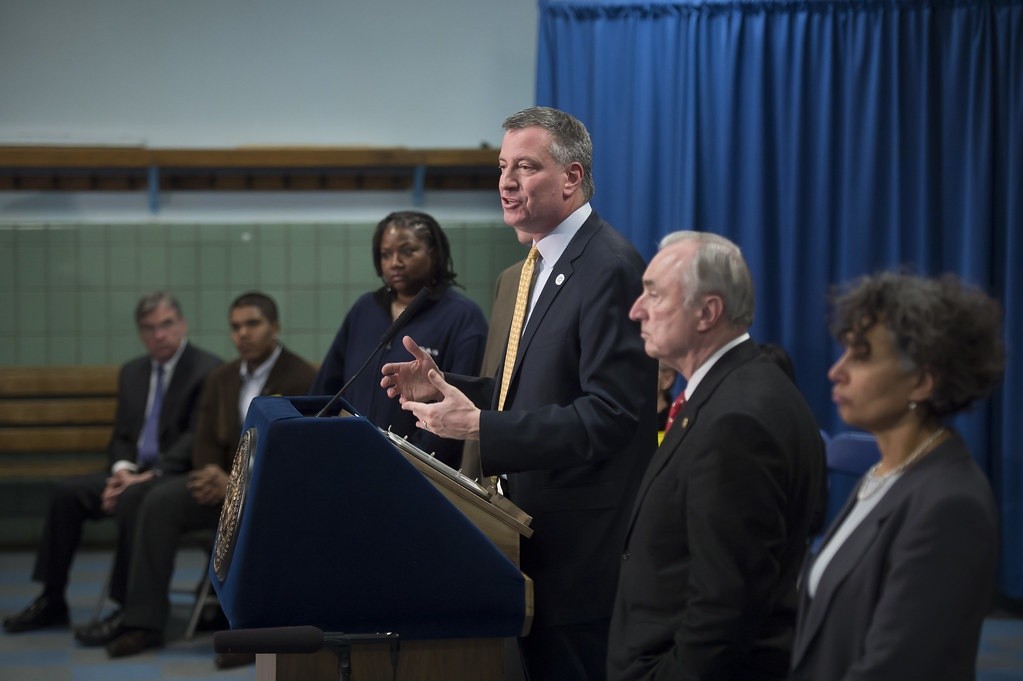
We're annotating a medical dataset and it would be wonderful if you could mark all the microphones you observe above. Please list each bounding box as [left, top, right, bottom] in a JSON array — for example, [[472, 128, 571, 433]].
[[380, 286, 432, 343]]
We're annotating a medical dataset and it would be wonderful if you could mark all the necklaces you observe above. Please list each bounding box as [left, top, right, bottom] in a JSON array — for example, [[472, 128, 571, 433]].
[[854, 421, 948, 503]]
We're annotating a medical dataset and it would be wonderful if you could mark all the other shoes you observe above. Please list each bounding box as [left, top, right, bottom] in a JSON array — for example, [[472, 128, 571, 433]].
[[215, 652, 256, 669]]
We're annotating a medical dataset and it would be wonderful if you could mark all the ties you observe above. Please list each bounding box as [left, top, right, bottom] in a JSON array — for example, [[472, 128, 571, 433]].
[[662, 392, 687, 436], [487, 244, 541, 484], [137, 366, 166, 468]]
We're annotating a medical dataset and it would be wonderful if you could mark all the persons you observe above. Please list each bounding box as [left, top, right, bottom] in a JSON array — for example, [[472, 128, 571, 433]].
[[1, 292, 229, 647], [603, 230, 821, 680], [378, 106, 660, 681], [785, 274, 1001, 679], [102, 291, 326, 672], [659, 354, 678, 439], [305, 211, 488, 463]]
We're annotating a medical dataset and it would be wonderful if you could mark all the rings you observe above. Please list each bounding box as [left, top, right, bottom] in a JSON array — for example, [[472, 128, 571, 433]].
[[422, 420, 429, 431]]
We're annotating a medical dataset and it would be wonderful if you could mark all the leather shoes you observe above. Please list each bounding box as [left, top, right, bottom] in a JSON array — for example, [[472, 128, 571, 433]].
[[107, 628, 166, 658], [75, 609, 126, 644], [3, 596, 69, 633]]
